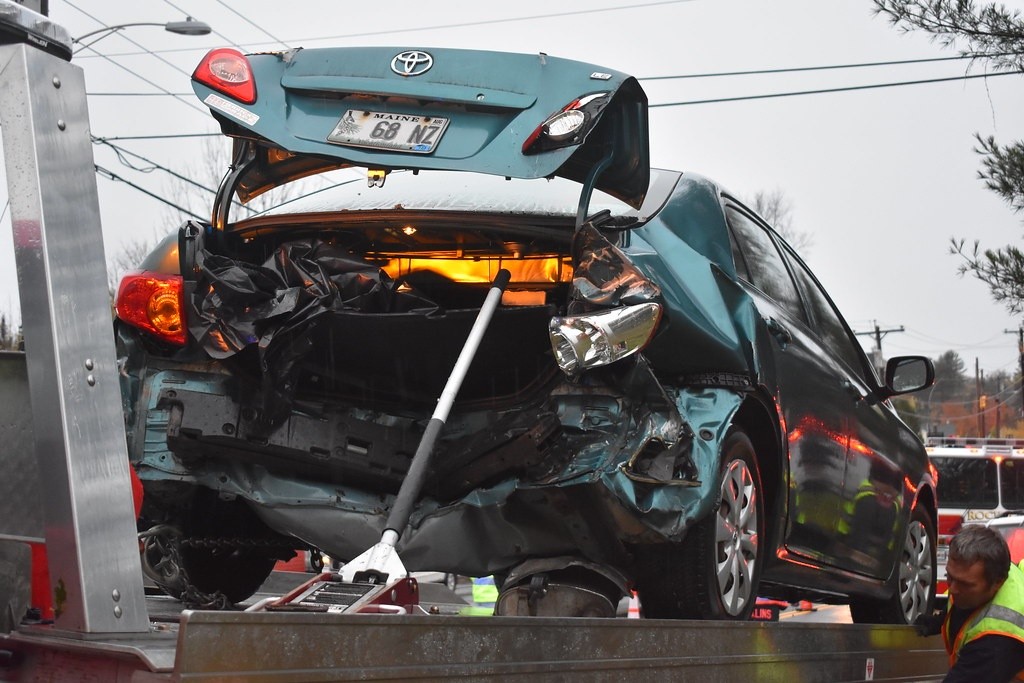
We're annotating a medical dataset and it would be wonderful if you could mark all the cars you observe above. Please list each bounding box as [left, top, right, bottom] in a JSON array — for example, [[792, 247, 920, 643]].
[[104, 42, 942, 640]]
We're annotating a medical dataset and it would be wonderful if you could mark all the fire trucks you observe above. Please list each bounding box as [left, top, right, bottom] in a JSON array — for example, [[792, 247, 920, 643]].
[[922, 443, 1024, 602]]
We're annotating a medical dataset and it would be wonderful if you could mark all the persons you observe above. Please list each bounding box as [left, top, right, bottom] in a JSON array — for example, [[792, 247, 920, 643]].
[[912, 526, 1023, 683]]
